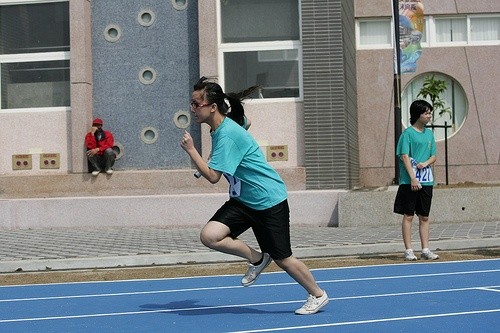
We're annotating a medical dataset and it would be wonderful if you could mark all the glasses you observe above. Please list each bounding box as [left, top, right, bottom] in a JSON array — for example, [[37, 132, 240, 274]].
[[190, 102, 212, 109]]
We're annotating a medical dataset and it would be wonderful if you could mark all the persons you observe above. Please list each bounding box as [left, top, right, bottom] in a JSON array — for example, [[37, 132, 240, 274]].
[[180, 77, 330, 315], [393, 100, 440, 261], [85, 118, 115, 176]]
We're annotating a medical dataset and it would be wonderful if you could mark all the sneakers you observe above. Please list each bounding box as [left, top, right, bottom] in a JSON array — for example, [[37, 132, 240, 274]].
[[92, 170, 100, 175], [421, 250, 439, 260], [105, 168, 113, 174], [295, 290, 329, 314], [241, 253, 272, 287], [405, 250, 417, 260]]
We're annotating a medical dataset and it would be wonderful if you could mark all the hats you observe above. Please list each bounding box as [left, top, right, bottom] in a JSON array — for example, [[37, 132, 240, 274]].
[[93, 118, 103, 124]]
